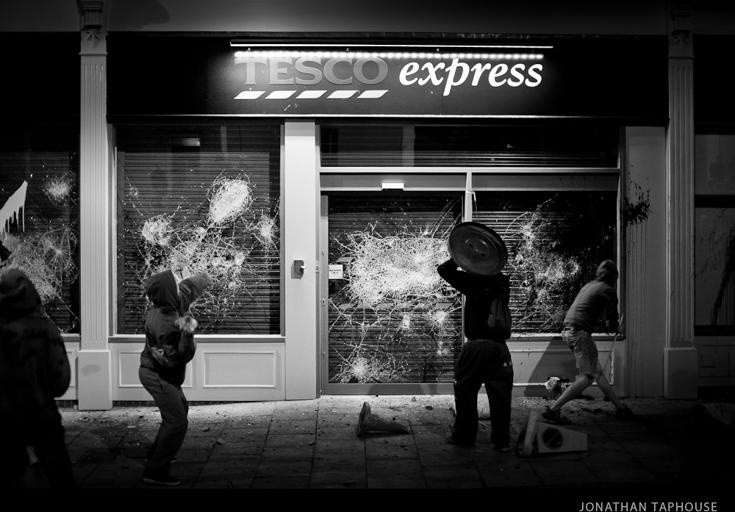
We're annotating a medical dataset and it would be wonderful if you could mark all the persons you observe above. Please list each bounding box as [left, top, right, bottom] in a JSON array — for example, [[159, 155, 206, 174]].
[[437, 256, 514, 452], [0, 268, 90, 512], [544, 259, 633, 420], [138, 265, 218, 487]]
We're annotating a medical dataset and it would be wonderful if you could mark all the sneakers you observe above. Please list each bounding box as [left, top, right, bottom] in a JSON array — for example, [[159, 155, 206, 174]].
[[614, 403, 634, 422], [144, 470, 181, 486], [541, 407, 566, 426]]
